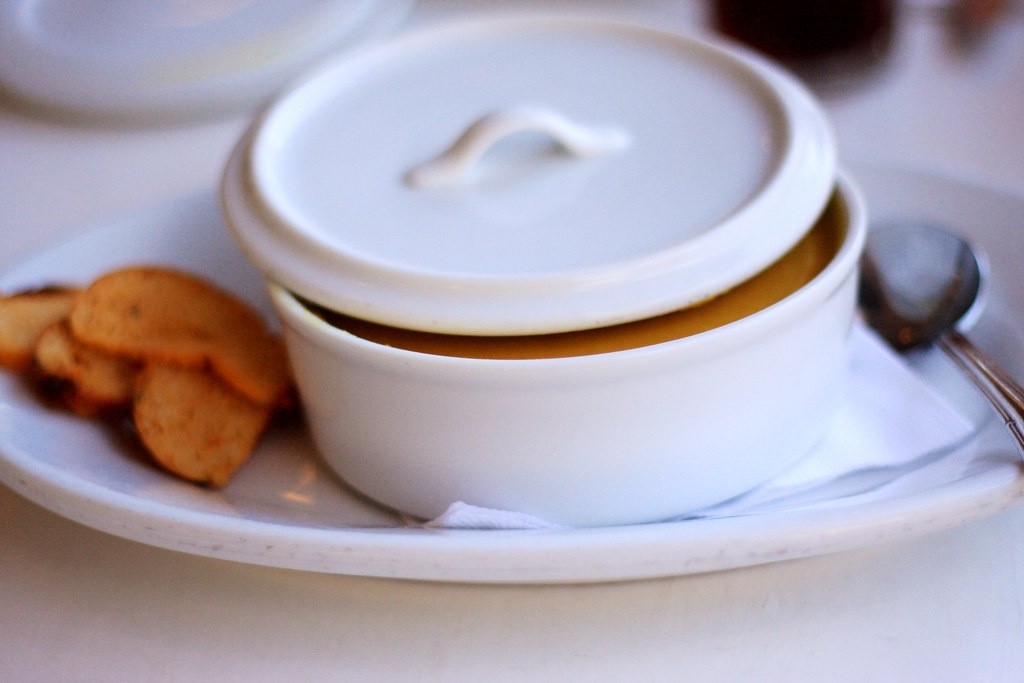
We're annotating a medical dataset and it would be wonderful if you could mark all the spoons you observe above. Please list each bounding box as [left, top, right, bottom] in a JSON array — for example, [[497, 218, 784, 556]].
[[859, 218, 1024, 456]]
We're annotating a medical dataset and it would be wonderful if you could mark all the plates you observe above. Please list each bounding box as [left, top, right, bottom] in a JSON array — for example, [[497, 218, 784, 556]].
[[1, 192, 1024, 585], [0, 0, 417, 124]]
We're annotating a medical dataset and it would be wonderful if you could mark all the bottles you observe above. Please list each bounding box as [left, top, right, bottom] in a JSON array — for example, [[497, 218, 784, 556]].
[[706, 0, 898, 78]]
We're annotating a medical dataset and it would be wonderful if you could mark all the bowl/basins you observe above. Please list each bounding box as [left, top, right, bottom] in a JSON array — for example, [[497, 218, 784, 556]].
[[265, 163, 867, 526]]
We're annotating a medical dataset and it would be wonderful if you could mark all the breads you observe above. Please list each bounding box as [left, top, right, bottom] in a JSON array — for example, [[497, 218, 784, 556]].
[[0, 268, 295, 490]]
